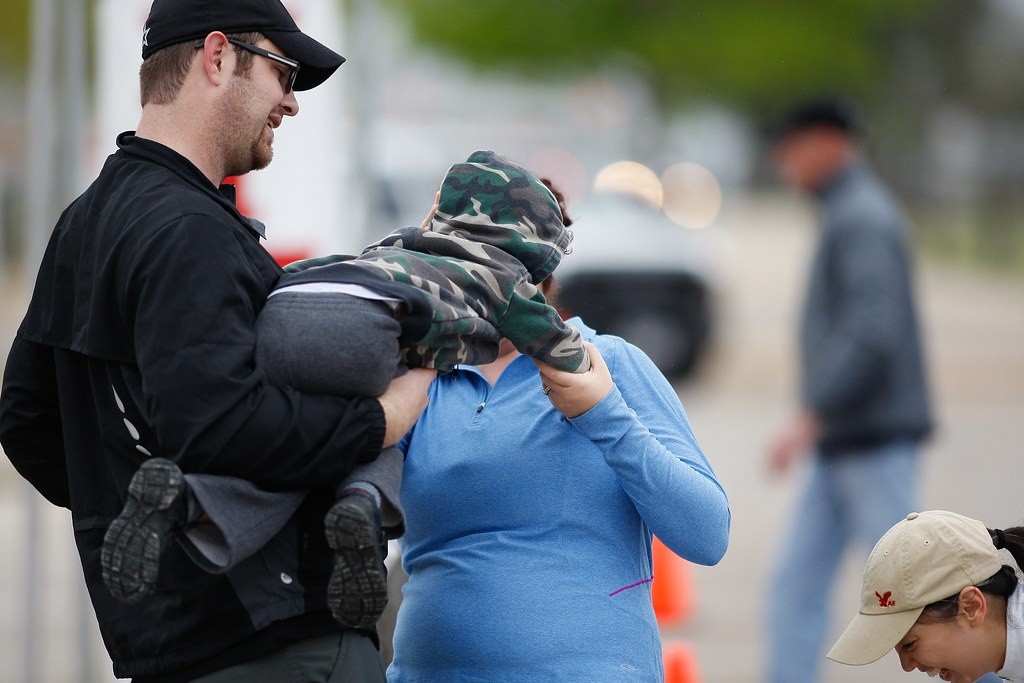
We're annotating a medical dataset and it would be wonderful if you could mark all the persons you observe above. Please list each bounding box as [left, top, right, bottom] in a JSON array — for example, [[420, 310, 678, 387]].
[[751, 99, 926, 681], [382, 273, 732, 683], [101, 152, 589, 628], [0, 0, 437, 683], [825, 509, 1024, 683]]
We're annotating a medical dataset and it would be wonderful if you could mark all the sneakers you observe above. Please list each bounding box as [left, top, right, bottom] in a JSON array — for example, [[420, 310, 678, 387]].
[[101, 459, 188, 604], [326, 493, 388, 630]]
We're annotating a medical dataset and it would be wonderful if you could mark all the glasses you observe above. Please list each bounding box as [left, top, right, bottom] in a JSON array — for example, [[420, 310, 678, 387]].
[[195, 35, 300, 94]]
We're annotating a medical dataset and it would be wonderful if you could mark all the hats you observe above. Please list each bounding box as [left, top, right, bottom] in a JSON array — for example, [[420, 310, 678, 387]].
[[826, 510, 1003, 667], [762, 100, 852, 136], [141, 0, 346, 92]]
